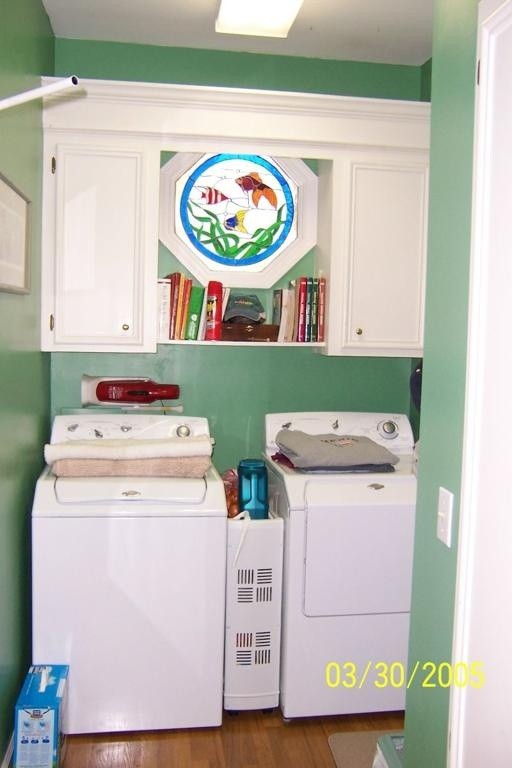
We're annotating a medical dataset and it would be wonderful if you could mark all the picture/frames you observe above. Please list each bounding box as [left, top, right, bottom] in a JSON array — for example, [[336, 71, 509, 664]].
[[0, 173, 34, 295]]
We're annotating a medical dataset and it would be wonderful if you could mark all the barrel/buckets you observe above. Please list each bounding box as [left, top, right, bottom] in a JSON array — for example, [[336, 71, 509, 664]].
[[238, 458, 270, 518]]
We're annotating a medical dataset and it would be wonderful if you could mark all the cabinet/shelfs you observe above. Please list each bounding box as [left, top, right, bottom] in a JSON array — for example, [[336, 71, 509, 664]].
[[42, 129, 159, 356], [315, 149, 427, 358]]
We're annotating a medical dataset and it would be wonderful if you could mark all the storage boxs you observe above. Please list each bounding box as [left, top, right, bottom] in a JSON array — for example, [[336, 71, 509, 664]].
[[15, 665, 69, 767]]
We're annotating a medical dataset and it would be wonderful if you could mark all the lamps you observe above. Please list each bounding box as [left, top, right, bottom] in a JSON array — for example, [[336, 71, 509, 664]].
[[215, 0, 304, 39]]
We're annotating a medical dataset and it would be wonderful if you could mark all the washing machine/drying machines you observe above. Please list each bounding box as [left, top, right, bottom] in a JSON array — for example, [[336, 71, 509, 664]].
[[262, 411, 419, 717], [31, 416, 227, 737]]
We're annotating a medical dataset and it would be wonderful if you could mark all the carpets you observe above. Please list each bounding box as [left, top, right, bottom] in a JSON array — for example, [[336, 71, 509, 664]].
[[329, 729, 402, 767]]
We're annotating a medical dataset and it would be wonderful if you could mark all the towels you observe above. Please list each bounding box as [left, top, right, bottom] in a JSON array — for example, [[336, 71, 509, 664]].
[[40, 434, 212, 479]]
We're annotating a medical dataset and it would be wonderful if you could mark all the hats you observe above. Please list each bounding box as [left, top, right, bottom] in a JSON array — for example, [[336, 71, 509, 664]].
[[223, 295, 266, 321]]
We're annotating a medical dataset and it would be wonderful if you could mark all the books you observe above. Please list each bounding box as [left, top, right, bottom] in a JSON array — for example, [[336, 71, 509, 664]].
[[157, 272, 327, 341]]
